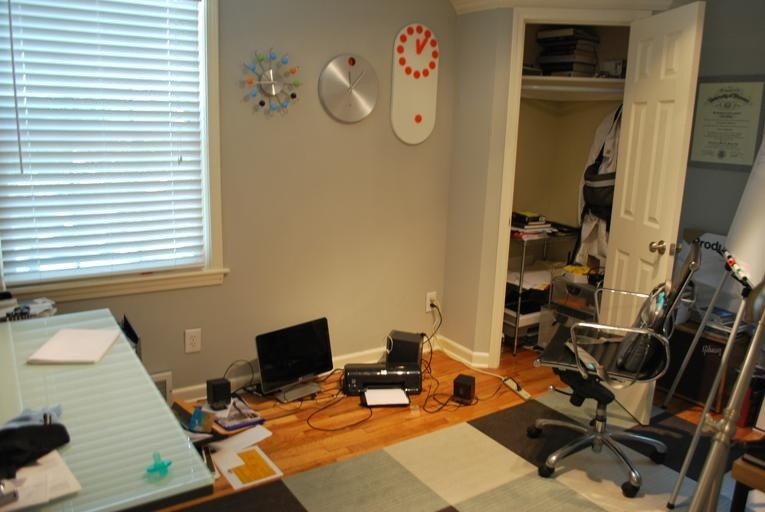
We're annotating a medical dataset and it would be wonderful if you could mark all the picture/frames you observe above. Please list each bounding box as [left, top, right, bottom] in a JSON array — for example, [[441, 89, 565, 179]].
[[692, 74, 764, 172]]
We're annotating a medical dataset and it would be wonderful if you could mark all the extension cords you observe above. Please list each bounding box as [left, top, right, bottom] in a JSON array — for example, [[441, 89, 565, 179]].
[[502, 376, 531, 401]]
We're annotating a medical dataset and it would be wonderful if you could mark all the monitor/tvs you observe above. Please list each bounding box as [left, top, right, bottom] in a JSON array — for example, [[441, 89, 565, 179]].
[[255, 317, 333, 404]]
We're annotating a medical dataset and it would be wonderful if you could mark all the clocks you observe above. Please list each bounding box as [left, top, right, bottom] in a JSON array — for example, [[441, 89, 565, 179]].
[[233, 21, 444, 146]]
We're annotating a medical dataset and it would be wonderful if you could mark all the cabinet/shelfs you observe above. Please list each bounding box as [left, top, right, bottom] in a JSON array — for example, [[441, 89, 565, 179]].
[[440, 1, 707, 369], [502, 225, 600, 358]]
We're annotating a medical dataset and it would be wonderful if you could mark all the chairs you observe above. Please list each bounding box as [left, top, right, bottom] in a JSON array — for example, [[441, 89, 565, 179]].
[[524, 238, 698, 502]]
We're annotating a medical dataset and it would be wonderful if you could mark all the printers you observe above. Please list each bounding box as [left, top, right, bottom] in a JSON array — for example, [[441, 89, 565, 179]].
[[344, 363, 421, 408]]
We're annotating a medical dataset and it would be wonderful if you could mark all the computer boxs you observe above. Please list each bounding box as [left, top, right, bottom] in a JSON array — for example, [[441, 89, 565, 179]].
[[386, 330, 423, 369]]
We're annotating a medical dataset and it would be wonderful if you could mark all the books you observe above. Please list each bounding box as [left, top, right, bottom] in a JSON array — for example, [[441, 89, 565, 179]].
[[536, 26, 597, 77], [27, 327, 121, 365], [512, 210, 551, 233], [699, 307, 747, 334]]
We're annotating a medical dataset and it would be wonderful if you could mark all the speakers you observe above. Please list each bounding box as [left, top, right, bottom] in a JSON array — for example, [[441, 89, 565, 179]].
[[207, 377, 231, 410], [452, 374, 475, 404]]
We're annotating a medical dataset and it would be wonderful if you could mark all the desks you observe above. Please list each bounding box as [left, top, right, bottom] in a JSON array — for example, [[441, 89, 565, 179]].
[[1, 305, 216, 509]]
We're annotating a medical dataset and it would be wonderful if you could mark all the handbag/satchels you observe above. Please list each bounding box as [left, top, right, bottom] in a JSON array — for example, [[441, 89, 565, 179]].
[[616, 281, 672, 372], [583, 162, 615, 206]]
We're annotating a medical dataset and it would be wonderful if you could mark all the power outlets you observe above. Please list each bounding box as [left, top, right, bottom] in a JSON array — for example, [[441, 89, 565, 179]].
[[183, 328, 203, 355]]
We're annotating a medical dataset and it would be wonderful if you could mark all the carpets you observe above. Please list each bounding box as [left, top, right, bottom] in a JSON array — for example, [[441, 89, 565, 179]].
[[171, 379, 765, 511]]
